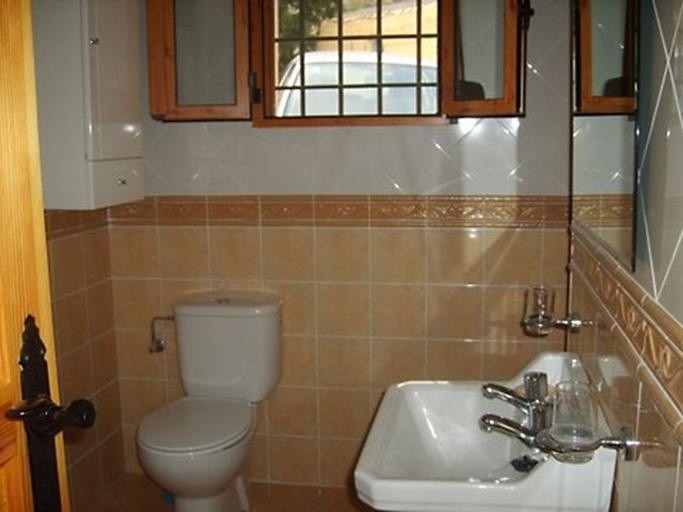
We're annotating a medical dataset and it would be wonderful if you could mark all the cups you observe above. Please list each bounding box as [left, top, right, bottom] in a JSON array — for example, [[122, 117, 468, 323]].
[[522, 283, 557, 338], [547, 380, 599, 467]]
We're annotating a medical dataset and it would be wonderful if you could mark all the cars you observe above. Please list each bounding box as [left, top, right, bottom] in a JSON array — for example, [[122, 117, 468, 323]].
[[275, 49, 438, 119]]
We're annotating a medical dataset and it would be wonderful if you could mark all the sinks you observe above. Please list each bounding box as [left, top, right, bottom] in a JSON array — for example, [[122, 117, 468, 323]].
[[353, 347, 619, 512]]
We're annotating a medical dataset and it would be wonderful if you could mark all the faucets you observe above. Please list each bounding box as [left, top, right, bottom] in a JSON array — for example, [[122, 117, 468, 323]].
[[477, 401, 553, 446], [481, 374, 549, 416]]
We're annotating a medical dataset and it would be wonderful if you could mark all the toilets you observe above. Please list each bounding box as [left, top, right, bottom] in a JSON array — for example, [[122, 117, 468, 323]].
[[134, 289, 285, 512]]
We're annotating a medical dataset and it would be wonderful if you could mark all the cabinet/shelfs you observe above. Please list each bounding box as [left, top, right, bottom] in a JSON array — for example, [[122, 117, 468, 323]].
[[30, 0, 146, 212]]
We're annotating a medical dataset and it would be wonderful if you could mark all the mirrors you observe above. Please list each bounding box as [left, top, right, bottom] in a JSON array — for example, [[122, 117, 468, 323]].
[[568, 1, 683, 354]]
